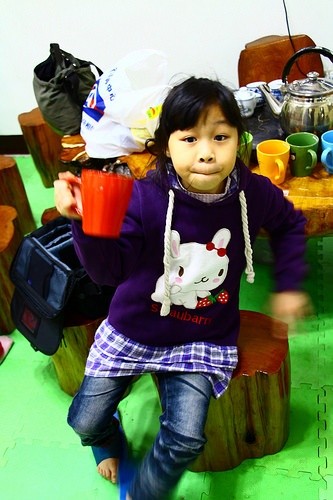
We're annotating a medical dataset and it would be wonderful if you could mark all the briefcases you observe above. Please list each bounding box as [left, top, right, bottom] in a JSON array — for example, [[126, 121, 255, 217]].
[[10, 216, 104, 356]]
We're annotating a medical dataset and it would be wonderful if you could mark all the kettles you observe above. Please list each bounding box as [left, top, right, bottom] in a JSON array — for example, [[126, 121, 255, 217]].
[[258, 47, 333, 138]]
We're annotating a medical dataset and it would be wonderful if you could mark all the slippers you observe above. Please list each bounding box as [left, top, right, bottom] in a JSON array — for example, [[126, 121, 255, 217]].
[[92, 409, 127, 486], [120, 459, 144, 500]]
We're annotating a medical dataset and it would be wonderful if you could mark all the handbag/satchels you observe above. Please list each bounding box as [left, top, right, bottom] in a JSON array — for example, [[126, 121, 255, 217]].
[[78, 69, 157, 160], [33, 39, 104, 135]]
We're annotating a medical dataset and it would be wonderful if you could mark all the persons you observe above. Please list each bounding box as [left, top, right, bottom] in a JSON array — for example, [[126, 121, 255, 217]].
[[53, 76, 309, 500]]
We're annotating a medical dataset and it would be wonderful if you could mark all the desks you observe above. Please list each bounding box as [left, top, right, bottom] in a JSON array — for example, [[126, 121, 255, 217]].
[[122, 153, 333, 265]]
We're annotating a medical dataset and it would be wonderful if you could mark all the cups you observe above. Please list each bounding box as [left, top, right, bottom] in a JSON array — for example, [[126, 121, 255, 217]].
[[237, 132, 254, 168], [62, 169, 134, 238], [234, 91, 256, 117], [246, 81, 267, 107], [267, 78, 289, 103], [256, 139, 290, 185], [320, 130, 333, 174], [285, 132, 320, 177]]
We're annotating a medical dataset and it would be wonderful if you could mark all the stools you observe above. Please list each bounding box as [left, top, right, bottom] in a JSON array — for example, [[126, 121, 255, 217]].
[[0, 205, 25, 335], [0, 154, 36, 233], [61, 132, 90, 176], [153, 309, 292, 472], [238, 33, 324, 87], [51, 316, 106, 396], [19, 108, 69, 189]]
